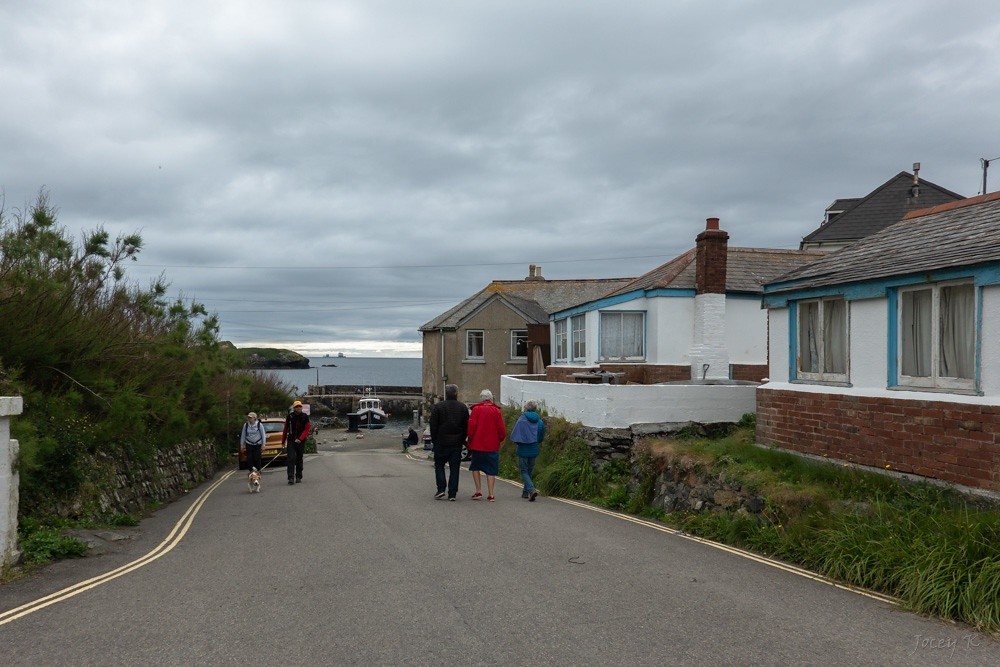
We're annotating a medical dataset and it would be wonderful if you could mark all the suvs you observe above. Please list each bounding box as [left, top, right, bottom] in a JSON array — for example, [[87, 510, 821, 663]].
[[239, 417, 289, 470]]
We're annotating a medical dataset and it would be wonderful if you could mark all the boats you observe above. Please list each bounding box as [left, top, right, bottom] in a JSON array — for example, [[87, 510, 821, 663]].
[[357, 357, 387, 429]]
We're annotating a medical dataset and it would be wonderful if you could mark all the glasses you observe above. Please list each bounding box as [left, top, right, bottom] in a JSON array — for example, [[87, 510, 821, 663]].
[[249, 417, 252, 418], [295, 406, 302, 408]]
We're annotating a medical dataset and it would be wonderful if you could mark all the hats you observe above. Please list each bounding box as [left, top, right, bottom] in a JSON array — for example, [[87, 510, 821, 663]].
[[293, 401, 302, 407], [247, 412, 257, 418]]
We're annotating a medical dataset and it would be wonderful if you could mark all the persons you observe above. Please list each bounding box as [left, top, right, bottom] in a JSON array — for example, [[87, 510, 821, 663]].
[[468, 389, 506, 501], [281, 400, 311, 485], [241, 412, 266, 474], [430, 384, 469, 501], [510, 399, 544, 501], [402, 427, 418, 453]]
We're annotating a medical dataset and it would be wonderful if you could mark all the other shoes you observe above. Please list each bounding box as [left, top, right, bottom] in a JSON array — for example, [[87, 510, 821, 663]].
[[448, 495, 455, 501], [296, 478, 302, 483], [288, 479, 294, 485], [522, 494, 528, 498], [435, 491, 445, 498], [529, 489, 537, 501]]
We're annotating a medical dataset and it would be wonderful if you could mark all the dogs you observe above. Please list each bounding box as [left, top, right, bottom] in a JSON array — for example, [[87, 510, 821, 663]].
[[247, 466, 261, 493]]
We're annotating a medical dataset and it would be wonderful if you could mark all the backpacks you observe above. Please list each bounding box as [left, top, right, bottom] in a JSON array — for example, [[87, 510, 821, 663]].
[[246, 419, 261, 436]]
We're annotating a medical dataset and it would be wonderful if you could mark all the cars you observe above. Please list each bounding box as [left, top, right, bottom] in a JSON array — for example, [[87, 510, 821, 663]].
[[460, 404, 478, 461]]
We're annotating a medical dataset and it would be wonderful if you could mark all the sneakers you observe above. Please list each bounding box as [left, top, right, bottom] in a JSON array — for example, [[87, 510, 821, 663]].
[[472, 492, 482, 499], [487, 495, 495, 501]]
